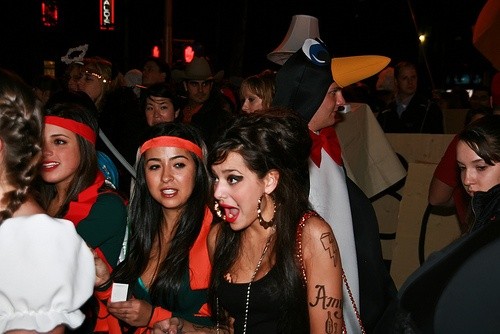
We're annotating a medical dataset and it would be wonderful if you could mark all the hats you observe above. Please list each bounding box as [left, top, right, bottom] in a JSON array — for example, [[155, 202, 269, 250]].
[[275, 37, 392, 124], [171, 60, 225, 87]]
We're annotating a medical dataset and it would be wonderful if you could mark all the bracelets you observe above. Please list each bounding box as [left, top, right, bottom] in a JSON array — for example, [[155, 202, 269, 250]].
[[94, 280, 112, 291], [148, 307, 172, 328]]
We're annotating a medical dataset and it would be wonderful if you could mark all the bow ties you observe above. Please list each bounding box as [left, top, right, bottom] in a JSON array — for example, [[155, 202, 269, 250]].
[[307, 126, 343, 168]]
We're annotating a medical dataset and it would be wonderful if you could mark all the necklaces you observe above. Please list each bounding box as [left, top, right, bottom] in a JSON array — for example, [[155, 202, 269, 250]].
[[216, 236, 281, 334]]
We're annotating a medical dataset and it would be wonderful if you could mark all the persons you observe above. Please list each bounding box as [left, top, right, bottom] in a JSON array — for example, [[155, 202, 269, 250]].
[[376, 62, 443, 134], [153, 110, 342, 334], [0, 68, 95, 334], [372, 106, 500, 334], [38, 91, 127, 334], [55, 55, 276, 154], [89, 122, 213, 334], [275, 38, 399, 334]]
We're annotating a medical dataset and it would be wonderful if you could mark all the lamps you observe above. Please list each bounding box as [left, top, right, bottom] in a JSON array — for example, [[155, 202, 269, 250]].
[[265, 14, 319, 66]]
